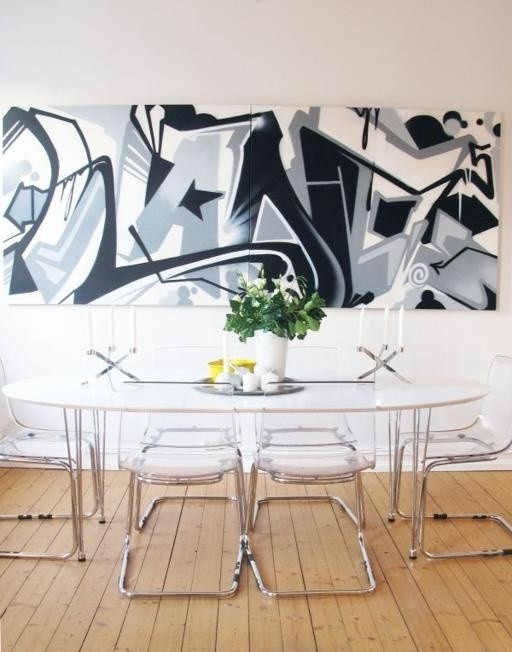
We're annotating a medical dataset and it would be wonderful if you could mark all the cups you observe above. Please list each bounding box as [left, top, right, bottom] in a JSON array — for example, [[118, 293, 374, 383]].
[[260, 373, 279, 392], [242, 374, 256, 391]]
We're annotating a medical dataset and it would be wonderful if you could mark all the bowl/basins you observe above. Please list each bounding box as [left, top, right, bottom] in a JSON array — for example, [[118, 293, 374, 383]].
[[207, 359, 256, 383]]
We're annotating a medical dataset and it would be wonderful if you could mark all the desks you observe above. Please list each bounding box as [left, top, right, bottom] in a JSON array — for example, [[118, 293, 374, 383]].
[[1, 373, 489, 561]]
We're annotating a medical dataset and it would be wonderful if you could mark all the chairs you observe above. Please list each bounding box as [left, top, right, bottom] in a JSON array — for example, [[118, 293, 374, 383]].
[[245, 380, 377, 599], [0, 357, 98, 561], [118, 381, 245, 599], [245, 346, 367, 534], [397, 355, 512, 558], [134, 346, 245, 535]]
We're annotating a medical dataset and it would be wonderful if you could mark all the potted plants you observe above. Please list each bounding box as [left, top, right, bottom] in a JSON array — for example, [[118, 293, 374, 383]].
[[223, 264, 327, 381]]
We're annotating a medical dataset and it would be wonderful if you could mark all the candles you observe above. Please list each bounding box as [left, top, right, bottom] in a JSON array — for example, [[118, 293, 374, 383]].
[[359, 307, 364, 347], [384, 306, 388, 345], [86, 307, 92, 351], [110, 305, 114, 346], [132, 306, 136, 348], [399, 305, 403, 347]]
